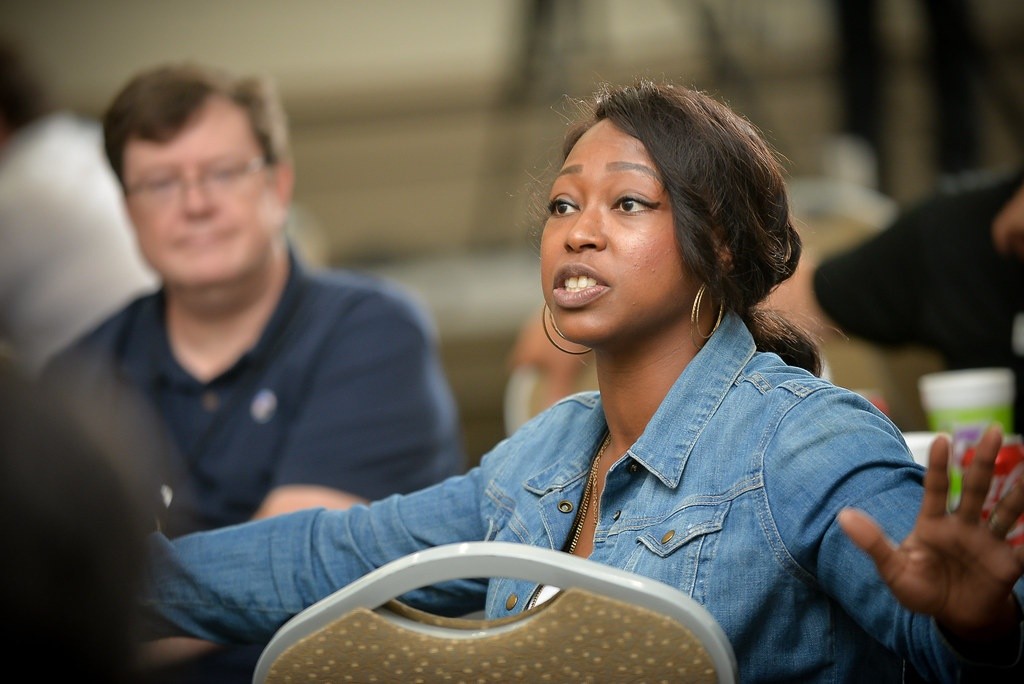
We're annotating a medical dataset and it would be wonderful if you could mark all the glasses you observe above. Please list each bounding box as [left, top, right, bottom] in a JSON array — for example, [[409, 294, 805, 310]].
[[122, 152, 272, 206]]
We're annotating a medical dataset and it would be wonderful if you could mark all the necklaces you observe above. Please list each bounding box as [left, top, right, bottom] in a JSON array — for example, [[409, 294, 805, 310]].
[[522, 432, 611, 611]]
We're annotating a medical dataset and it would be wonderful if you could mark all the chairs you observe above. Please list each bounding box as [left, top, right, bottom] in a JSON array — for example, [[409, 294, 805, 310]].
[[255, 539, 739, 684]]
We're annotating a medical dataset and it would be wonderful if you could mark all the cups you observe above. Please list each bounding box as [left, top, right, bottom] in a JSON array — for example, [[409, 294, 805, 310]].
[[918, 367, 1014, 511]]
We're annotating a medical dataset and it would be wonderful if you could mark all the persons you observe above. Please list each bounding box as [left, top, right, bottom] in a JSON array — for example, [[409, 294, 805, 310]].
[[132, 82, 1024, 684], [0, 41, 160, 371], [0, 348, 149, 684], [29, 64, 489, 684], [812, 170, 1024, 438]]
